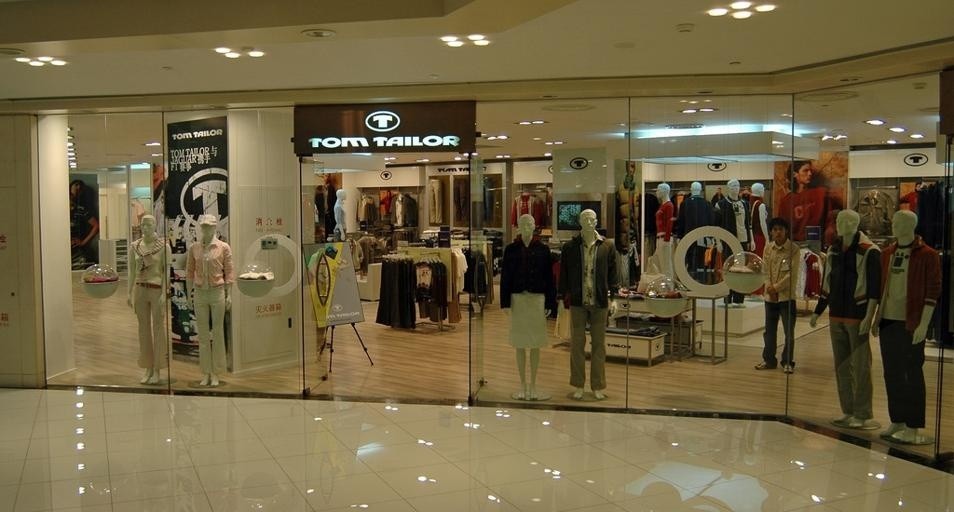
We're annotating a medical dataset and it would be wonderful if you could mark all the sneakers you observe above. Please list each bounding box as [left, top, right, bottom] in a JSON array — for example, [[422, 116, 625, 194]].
[[784, 364, 794, 373], [755, 362, 767, 369]]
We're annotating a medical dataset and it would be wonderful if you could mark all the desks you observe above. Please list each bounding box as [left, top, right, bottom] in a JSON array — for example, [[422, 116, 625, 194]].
[[605, 290, 693, 362]]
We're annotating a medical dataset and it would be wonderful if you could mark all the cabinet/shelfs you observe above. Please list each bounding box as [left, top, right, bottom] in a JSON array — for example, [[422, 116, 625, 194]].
[[340, 168, 426, 232], [614, 316, 703, 351], [513, 160, 553, 237], [356, 264, 385, 300], [583, 327, 667, 368], [847, 150, 953, 242], [382, 251, 417, 264]]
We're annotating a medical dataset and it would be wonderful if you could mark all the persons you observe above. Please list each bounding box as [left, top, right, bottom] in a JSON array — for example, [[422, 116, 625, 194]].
[[810, 210, 882, 427], [901, 183, 921, 211], [778, 161, 836, 251], [676, 181, 712, 245], [334, 189, 347, 242], [69, 180, 99, 270], [186, 215, 233, 386], [127, 214, 171, 384], [711, 188, 724, 206], [655, 183, 673, 274], [714, 179, 755, 309], [756, 218, 800, 373], [871, 210, 941, 443], [748, 183, 770, 297], [559, 210, 619, 399], [500, 215, 554, 399], [315, 173, 336, 233], [617, 160, 641, 287]]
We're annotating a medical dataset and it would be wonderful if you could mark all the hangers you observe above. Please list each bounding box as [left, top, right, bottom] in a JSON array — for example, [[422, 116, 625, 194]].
[[420, 251, 444, 268]]
